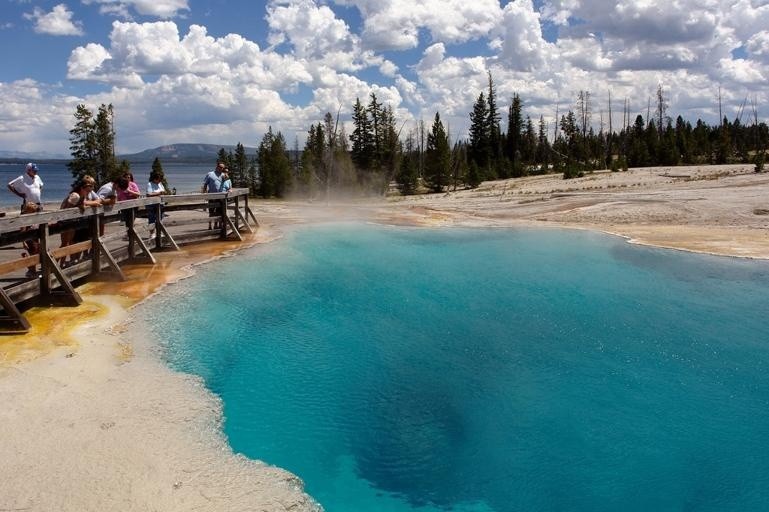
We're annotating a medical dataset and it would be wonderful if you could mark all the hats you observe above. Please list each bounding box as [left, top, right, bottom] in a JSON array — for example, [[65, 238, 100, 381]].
[[25, 163, 40, 171]]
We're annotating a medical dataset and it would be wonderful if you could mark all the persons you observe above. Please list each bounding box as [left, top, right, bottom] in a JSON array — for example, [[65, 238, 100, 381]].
[[218, 165, 234, 229], [57, 179, 95, 266], [19, 201, 41, 277], [74, 176, 103, 260], [117, 172, 141, 242], [89, 176, 129, 258], [7, 161, 44, 207], [202, 162, 230, 230], [145, 172, 169, 239]]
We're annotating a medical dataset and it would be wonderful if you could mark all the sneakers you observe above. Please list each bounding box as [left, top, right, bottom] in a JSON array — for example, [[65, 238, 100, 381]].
[[149, 233, 157, 240], [26, 257, 89, 277]]
